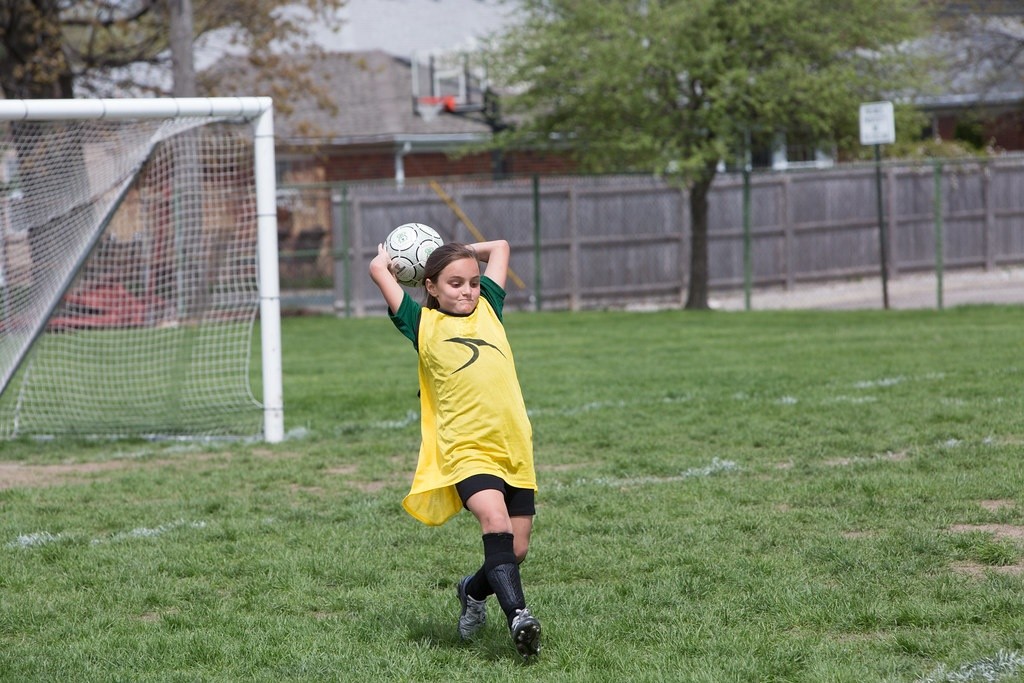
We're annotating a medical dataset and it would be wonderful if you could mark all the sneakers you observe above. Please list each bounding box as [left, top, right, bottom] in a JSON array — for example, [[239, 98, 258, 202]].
[[509, 608, 542, 662], [456, 576, 489, 645]]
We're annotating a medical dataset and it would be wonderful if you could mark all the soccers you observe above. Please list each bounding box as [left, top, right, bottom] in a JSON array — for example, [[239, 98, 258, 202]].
[[382, 222, 445, 288]]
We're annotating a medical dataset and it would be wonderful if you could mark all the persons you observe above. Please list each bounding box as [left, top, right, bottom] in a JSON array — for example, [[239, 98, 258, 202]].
[[370, 239, 542, 659]]
